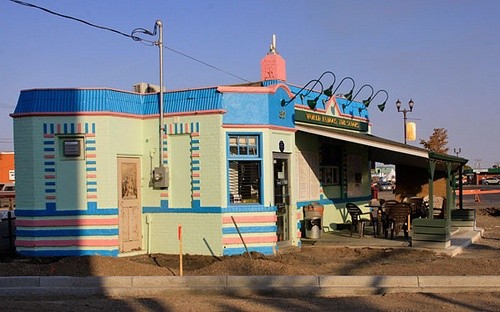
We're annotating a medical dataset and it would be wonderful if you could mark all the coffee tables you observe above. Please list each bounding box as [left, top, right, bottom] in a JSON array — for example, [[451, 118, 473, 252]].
[[364, 205, 394, 239]]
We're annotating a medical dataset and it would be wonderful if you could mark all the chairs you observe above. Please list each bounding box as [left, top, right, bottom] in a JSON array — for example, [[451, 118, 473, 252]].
[[346, 203, 377, 238], [384, 202, 413, 241]]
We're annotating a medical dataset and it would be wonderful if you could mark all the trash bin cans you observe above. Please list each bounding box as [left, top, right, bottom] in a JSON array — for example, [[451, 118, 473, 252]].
[[305, 216, 322, 239]]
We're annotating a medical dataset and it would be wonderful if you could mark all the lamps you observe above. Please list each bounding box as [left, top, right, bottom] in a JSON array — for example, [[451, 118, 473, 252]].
[[363, 100, 369, 107], [344, 93, 352, 101], [396, 99, 401, 108], [409, 100, 414, 108], [307, 99, 316, 110], [324, 88, 332, 96], [378, 104, 384, 111]]
